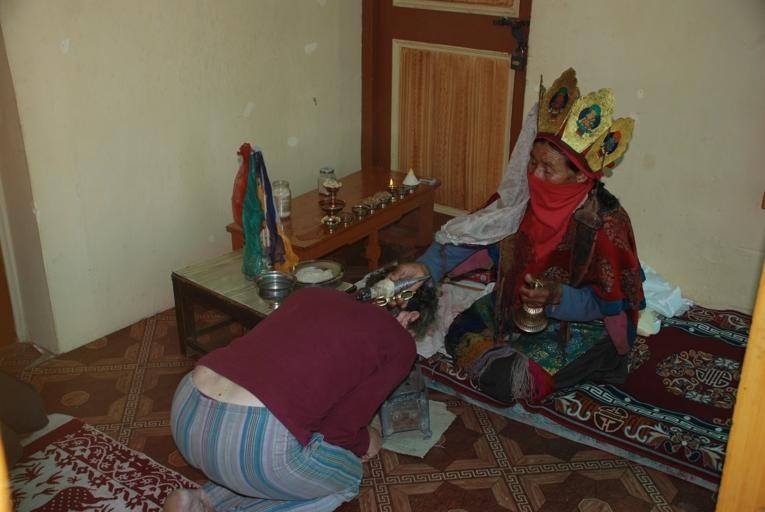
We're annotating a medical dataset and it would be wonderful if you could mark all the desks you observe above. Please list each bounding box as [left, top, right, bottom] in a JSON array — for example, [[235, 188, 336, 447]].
[[275, 166, 443, 278], [172, 250, 310, 360]]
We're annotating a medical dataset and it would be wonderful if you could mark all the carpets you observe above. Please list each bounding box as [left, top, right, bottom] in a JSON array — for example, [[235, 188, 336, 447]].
[[8, 410, 203, 512]]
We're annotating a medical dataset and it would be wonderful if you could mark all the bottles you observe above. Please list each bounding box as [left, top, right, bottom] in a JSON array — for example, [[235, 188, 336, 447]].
[[272, 179, 292, 219], [317, 166, 335, 196]]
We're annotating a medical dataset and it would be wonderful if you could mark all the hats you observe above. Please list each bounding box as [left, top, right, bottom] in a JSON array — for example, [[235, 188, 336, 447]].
[[537, 68, 635, 177]]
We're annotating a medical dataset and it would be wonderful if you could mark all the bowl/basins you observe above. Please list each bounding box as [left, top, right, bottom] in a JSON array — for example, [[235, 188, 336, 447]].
[[291, 259, 345, 288], [255, 270, 297, 301]]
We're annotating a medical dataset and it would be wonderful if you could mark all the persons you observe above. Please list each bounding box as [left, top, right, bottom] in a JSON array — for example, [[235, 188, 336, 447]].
[[161, 265, 444, 512], [373, 138, 650, 404]]
